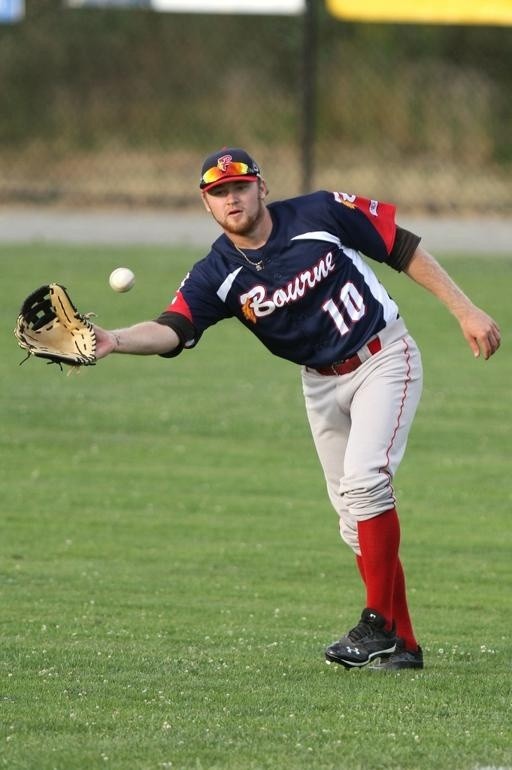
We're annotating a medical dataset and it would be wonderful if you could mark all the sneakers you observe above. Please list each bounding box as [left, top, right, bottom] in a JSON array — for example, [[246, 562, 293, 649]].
[[369, 644, 423, 671], [325, 608, 397, 669]]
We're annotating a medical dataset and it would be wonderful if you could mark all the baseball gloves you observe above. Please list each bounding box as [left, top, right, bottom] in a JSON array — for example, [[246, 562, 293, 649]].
[[13, 283, 98, 366]]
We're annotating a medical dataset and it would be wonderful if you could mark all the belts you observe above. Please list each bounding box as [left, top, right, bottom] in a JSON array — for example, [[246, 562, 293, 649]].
[[300, 336, 382, 378]]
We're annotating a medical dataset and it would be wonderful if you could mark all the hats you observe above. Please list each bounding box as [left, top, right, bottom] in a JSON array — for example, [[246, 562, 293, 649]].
[[199, 147, 262, 195]]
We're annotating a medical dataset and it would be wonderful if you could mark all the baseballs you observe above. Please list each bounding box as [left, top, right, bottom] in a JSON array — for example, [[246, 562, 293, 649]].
[[107, 267, 133, 292]]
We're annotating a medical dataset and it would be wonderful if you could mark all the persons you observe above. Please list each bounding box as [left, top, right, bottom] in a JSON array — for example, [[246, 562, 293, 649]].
[[13, 147, 502, 668]]
